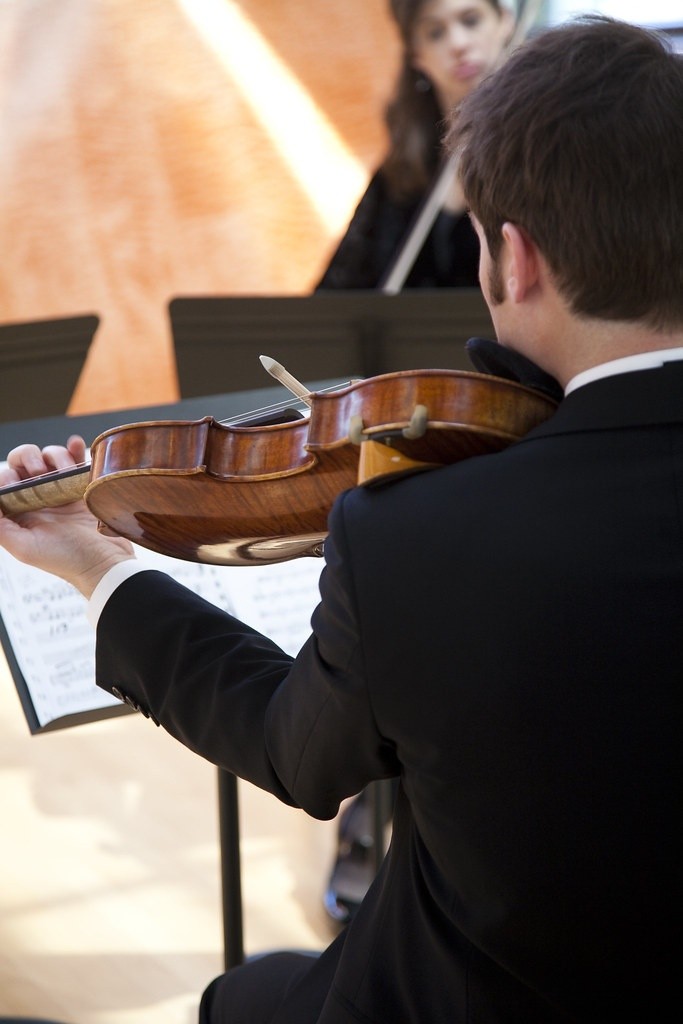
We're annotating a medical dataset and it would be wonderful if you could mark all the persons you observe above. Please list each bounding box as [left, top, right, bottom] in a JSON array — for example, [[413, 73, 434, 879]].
[[0, 0, 682, 1024]]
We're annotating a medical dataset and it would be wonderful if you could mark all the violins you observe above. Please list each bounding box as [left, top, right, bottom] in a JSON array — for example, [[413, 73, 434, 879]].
[[0, 337, 560, 566]]
